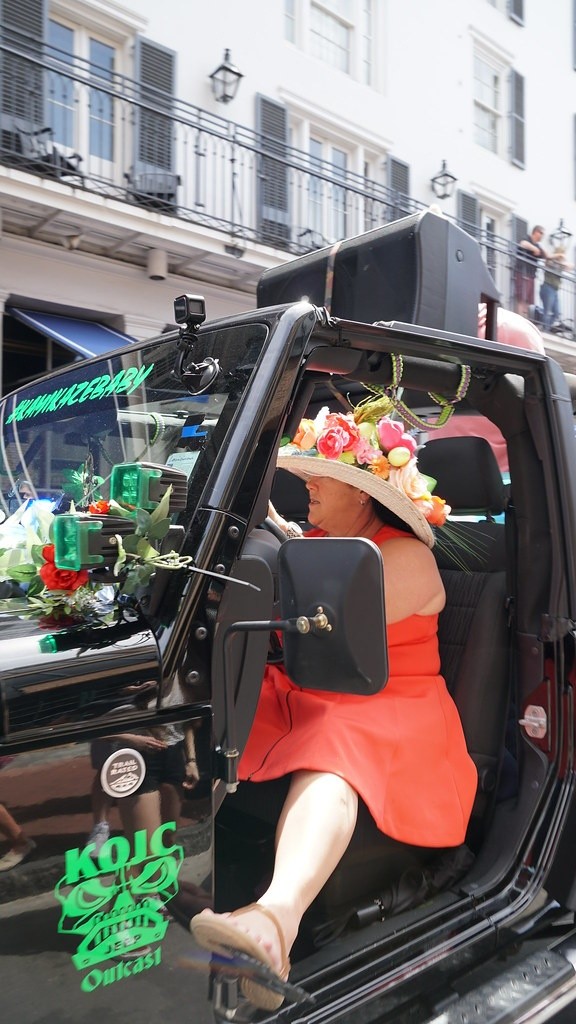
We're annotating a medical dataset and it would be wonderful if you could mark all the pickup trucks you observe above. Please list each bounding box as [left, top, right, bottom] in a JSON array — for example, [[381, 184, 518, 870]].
[[0, 208, 575, 1024]]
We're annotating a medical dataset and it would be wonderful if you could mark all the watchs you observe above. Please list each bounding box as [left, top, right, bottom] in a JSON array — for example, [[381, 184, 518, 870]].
[[285, 520, 303, 539]]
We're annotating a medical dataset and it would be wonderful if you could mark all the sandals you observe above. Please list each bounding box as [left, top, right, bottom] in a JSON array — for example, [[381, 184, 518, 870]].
[[191, 903, 291, 1011]]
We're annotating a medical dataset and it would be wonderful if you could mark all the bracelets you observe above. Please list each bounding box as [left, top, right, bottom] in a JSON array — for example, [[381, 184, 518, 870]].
[[186, 757, 196, 762]]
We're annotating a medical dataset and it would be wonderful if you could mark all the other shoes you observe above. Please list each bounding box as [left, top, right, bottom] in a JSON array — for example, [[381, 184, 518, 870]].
[[124, 893, 169, 959], [0, 839, 37, 873], [84, 821, 110, 858]]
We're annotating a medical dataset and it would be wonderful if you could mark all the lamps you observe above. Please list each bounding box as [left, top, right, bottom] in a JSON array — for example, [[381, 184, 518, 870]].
[[549, 217, 574, 251], [431, 157, 459, 199], [211, 46, 244, 104]]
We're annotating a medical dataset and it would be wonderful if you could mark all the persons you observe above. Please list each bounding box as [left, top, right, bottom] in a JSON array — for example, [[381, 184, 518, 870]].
[[17, 472, 36, 593], [513, 221, 547, 319], [90, 663, 198, 962], [191, 408, 477, 1009], [0, 802, 38, 873], [424, 300, 545, 522], [538, 244, 574, 334]]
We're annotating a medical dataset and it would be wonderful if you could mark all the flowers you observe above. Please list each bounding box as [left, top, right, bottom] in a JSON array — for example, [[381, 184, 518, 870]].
[[8, 485, 262, 617], [290, 405, 452, 526]]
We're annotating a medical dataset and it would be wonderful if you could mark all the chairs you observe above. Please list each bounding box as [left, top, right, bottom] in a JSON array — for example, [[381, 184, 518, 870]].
[[221, 434, 517, 906], [124, 172, 182, 216], [12, 120, 86, 188], [271, 465, 315, 532], [298, 229, 331, 254]]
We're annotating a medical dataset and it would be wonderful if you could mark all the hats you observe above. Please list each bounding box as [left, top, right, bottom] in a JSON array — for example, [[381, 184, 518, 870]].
[[275, 406, 451, 549]]
